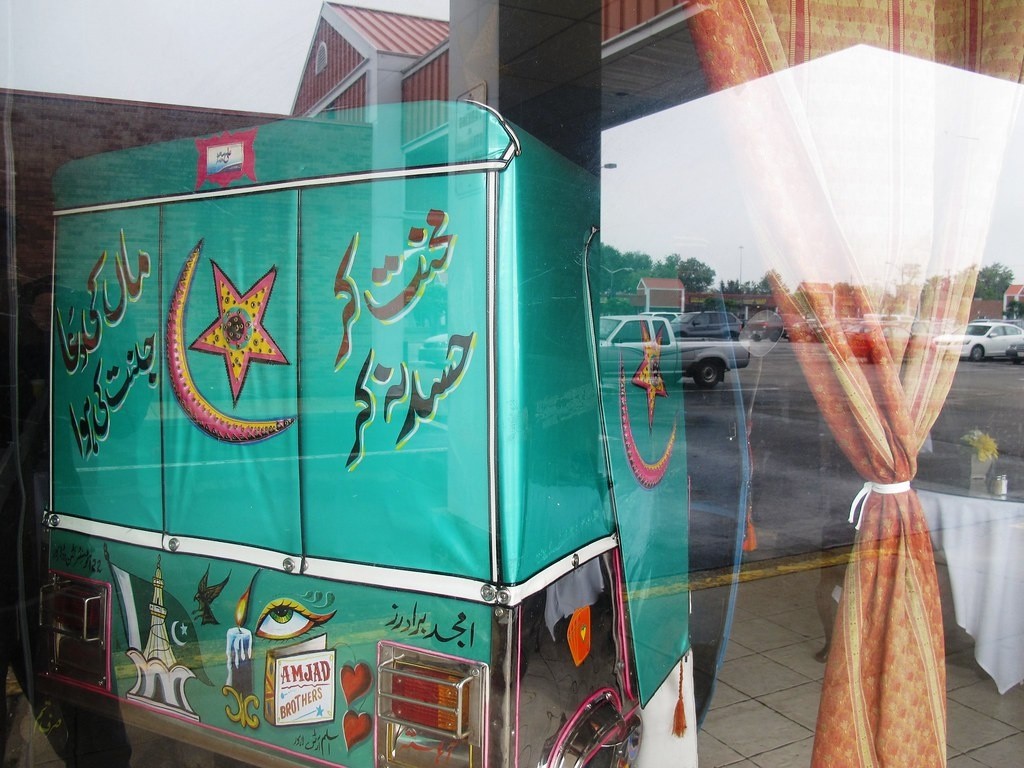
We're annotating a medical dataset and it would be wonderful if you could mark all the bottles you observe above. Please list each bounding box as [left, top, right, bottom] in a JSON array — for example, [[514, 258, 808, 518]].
[[1002, 475, 1008, 495], [996, 475, 1002, 495]]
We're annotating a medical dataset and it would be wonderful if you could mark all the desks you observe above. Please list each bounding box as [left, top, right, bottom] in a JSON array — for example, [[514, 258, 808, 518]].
[[814, 453, 1024, 695]]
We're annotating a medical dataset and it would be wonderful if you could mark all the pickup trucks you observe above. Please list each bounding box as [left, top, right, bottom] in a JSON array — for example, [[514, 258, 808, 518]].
[[600, 314, 753, 389]]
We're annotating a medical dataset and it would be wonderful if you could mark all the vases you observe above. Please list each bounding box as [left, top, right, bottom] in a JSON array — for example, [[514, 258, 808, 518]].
[[957, 445, 992, 479]]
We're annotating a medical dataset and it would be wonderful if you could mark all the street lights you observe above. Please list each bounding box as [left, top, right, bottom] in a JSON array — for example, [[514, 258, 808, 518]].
[[599, 265, 635, 300]]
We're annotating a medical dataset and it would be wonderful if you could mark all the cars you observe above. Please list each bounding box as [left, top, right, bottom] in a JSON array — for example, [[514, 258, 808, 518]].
[[639, 309, 1024, 365]]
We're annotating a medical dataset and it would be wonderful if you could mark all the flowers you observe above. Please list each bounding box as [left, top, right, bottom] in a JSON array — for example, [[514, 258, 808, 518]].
[[960, 428, 999, 462]]
